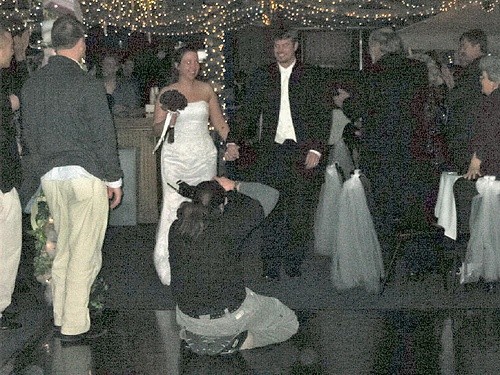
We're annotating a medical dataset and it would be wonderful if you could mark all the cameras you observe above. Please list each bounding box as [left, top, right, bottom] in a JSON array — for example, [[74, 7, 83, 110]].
[[178, 182, 196, 198]]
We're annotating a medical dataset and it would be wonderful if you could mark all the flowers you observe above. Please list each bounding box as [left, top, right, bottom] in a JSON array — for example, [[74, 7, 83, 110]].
[[160, 89, 188, 144]]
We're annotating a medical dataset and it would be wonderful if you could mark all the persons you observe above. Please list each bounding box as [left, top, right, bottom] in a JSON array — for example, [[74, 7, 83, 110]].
[[409, 27, 500, 179], [98, 53, 138, 116], [0, 26, 24, 330], [222, 25, 334, 280], [151, 46, 240, 288], [332, 25, 430, 283], [166, 175, 300, 357], [19, 14, 125, 342], [3, 20, 41, 214]]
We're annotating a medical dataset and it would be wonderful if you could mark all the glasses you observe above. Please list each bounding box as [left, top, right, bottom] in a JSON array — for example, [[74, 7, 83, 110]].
[[479, 76, 485, 81]]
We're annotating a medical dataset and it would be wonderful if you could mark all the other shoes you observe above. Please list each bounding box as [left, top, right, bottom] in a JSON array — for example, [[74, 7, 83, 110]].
[[206, 330, 249, 356], [185, 330, 208, 355], [52, 325, 63, 337], [483, 282, 497, 293], [59, 326, 108, 341], [286, 267, 301, 278], [1, 311, 15, 319], [0, 318, 22, 330], [385, 274, 396, 286], [264, 270, 281, 282], [408, 272, 418, 283]]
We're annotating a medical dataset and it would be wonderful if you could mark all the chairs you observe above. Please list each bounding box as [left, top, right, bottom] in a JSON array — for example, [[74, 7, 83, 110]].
[[353, 170, 448, 298], [450, 177, 500, 293]]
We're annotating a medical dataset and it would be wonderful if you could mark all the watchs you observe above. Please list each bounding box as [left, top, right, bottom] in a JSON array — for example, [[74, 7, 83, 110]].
[[233, 181, 240, 194]]
